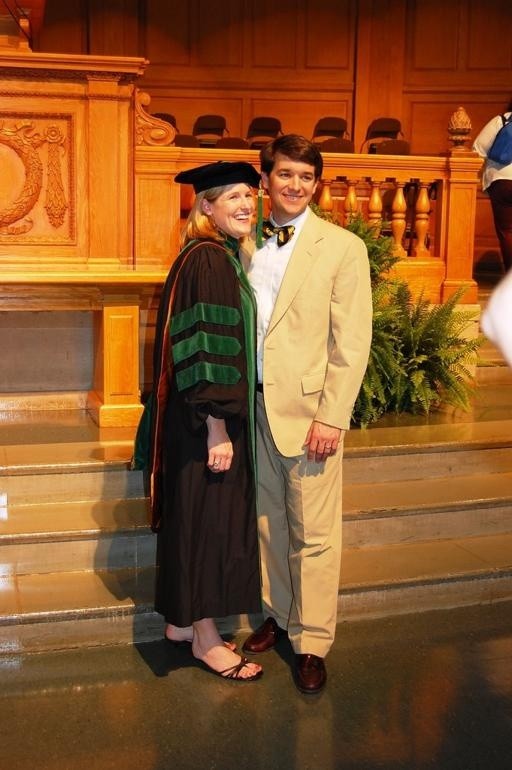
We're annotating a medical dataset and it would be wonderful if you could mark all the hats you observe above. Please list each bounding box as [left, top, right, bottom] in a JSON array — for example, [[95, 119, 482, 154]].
[[175, 161, 263, 249]]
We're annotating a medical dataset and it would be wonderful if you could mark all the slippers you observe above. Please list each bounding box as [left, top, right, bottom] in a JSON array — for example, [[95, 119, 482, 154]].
[[164, 633, 237, 651], [192, 651, 264, 681]]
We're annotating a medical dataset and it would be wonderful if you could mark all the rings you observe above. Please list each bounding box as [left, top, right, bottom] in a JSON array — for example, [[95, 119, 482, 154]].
[[215, 461, 220, 465], [325, 445, 332, 450]]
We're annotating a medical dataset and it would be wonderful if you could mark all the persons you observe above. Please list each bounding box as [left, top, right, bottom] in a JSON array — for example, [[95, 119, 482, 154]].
[[472, 103, 512, 273], [244, 134, 374, 694], [131, 160, 263, 681]]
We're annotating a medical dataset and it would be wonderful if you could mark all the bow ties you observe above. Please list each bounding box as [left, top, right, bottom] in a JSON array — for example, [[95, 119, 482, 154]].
[[255, 220, 295, 247]]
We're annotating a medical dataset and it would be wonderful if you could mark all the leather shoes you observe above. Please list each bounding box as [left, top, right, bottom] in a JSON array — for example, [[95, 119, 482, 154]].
[[242, 616, 287, 655], [292, 654, 327, 694]]
